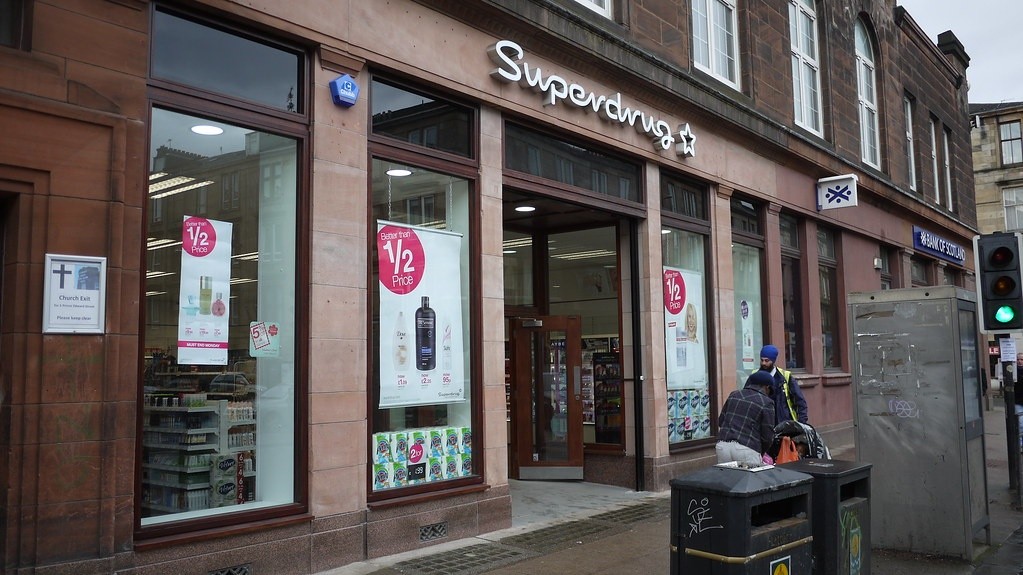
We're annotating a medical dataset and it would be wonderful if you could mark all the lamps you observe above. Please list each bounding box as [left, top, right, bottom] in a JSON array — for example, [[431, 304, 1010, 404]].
[[969, 114, 981, 128]]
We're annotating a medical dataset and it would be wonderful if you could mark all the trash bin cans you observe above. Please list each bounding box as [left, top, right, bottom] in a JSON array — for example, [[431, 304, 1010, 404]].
[[667, 460, 815, 575], [775, 459, 872, 574]]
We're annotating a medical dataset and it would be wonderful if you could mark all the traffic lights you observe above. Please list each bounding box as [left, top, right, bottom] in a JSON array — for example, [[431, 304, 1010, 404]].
[[974, 229, 1023, 332]]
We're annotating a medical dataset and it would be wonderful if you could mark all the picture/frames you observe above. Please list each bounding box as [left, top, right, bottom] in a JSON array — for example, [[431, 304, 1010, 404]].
[[41, 253, 107, 337]]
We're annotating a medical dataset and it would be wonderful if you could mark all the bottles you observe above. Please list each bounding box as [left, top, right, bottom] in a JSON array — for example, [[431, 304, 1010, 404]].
[[416, 296, 436, 371], [596, 366, 618, 398], [212, 292, 225, 316]]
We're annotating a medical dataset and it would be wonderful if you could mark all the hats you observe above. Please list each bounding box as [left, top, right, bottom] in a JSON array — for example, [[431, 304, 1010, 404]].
[[746, 370, 777, 395], [758, 345, 778, 364]]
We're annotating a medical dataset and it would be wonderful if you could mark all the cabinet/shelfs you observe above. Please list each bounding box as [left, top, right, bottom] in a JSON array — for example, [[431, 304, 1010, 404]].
[[140, 371, 257, 513], [505, 334, 622, 446]]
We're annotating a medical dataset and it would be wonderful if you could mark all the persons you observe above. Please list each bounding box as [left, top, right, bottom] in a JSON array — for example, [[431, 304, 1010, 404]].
[[743, 345, 808, 426], [714, 370, 776, 469], [795, 443, 808, 461], [1015, 353, 1023, 455]]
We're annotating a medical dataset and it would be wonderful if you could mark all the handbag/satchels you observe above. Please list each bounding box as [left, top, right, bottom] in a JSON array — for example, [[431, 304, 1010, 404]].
[[775, 437, 799, 466]]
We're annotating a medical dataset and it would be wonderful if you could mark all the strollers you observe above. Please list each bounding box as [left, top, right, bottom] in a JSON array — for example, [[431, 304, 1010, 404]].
[[767, 420, 832, 463]]
